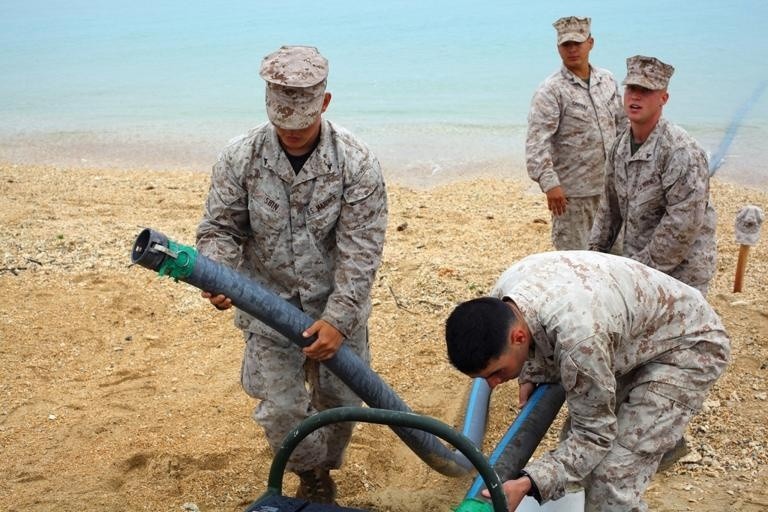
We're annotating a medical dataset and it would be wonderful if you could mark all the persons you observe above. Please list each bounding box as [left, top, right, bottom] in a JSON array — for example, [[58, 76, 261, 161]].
[[446, 248, 730, 512], [525, 15, 630, 255], [588, 55, 719, 296], [196, 43, 388, 512]]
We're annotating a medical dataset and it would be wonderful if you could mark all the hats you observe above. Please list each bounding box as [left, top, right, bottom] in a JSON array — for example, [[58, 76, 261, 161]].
[[621, 55, 676, 91], [734, 205, 764, 246], [551, 16, 592, 46], [258, 46, 329, 130]]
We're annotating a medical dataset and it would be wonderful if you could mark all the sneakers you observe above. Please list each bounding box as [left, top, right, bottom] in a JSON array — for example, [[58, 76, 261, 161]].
[[295, 467, 337, 503], [657, 436, 688, 472]]
